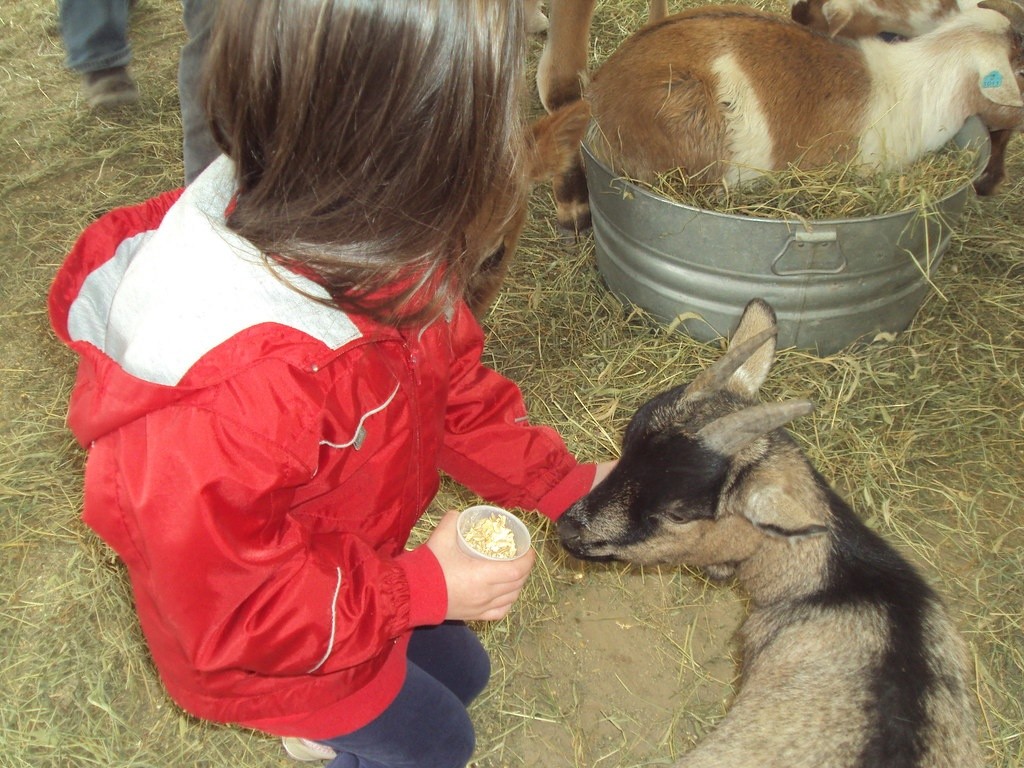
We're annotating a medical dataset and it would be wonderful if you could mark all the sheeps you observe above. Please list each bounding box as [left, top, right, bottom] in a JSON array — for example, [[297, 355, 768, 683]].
[[551, 298, 992, 768], [517, 0, 1024, 258]]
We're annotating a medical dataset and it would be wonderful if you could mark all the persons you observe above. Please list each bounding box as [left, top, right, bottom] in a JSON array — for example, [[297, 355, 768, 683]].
[[58, 0, 223, 186], [48, 0, 622, 768]]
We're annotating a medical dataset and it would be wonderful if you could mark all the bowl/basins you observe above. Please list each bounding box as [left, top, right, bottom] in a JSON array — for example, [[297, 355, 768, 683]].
[[579, 113, 992, 361]]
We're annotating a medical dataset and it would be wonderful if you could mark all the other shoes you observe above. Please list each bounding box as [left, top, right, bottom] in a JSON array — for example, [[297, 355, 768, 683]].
[[282, 735, 338, 761], [86, 68, 137, 109]]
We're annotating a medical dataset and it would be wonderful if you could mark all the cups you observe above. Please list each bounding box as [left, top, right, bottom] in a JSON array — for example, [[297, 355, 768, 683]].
[[457, 506, 530, 562]]
